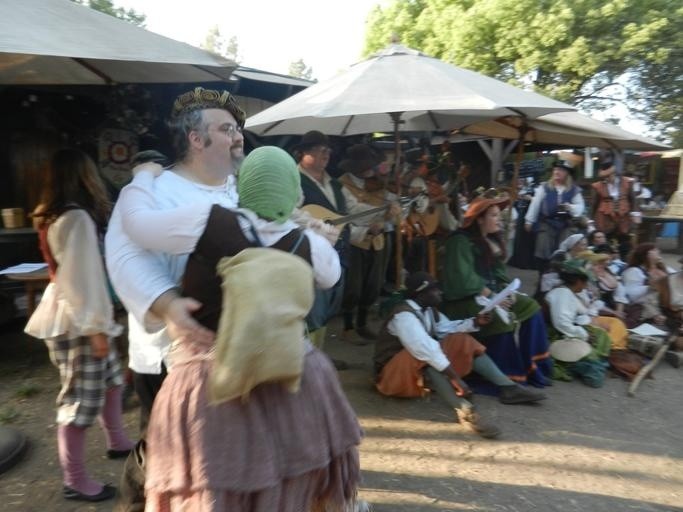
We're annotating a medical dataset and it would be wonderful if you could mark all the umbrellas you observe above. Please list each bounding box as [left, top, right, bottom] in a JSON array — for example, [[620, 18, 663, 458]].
[[460, 88, 674, 253], [242, 43, 575, 293], [0, 0, 240, 92]]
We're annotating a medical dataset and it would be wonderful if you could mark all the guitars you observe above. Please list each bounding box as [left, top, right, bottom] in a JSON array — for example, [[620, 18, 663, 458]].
[[299, 196, 402, 250], [407, 165, 468, 236]]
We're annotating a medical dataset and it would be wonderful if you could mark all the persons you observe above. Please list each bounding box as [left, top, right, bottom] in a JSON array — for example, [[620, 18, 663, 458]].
[[21, 143, 138, 503], [295, 130, 682, 439], [102, 84, 340, 512], [116, 144, 365, 510]]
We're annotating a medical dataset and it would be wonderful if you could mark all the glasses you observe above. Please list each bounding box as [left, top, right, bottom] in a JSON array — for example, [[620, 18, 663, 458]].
[[198, 125, 241, 137]]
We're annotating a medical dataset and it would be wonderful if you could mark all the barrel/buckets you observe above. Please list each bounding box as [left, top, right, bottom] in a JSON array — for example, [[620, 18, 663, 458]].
[[1, 206, 26, 229]]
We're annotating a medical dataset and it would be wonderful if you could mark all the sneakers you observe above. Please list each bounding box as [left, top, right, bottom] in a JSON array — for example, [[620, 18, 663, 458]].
[[457, 408, 501, 436], [500, 385, 549, 403], [341, 327, 377, 341]]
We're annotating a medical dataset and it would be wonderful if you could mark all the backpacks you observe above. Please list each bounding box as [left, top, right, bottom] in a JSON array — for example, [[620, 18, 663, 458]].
[[206, 209, 309, 402]]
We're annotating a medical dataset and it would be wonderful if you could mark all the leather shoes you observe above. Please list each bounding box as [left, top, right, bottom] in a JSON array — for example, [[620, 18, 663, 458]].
[[108, 449, 130, 459], [63, 483, 117, 502]]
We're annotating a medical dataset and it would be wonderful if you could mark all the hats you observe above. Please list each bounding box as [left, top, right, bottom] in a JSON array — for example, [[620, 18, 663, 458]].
[[171, 87, 246, 127], [289, 131, 336, 146], [564, 260, 592, 279], [462, 197, 511, 230], [599, 163, 615, 177], [403, 271, 442, 297], [551, 160, 576, 180], [337, 143, 389, 173], [414, 155, 434, 164]]
